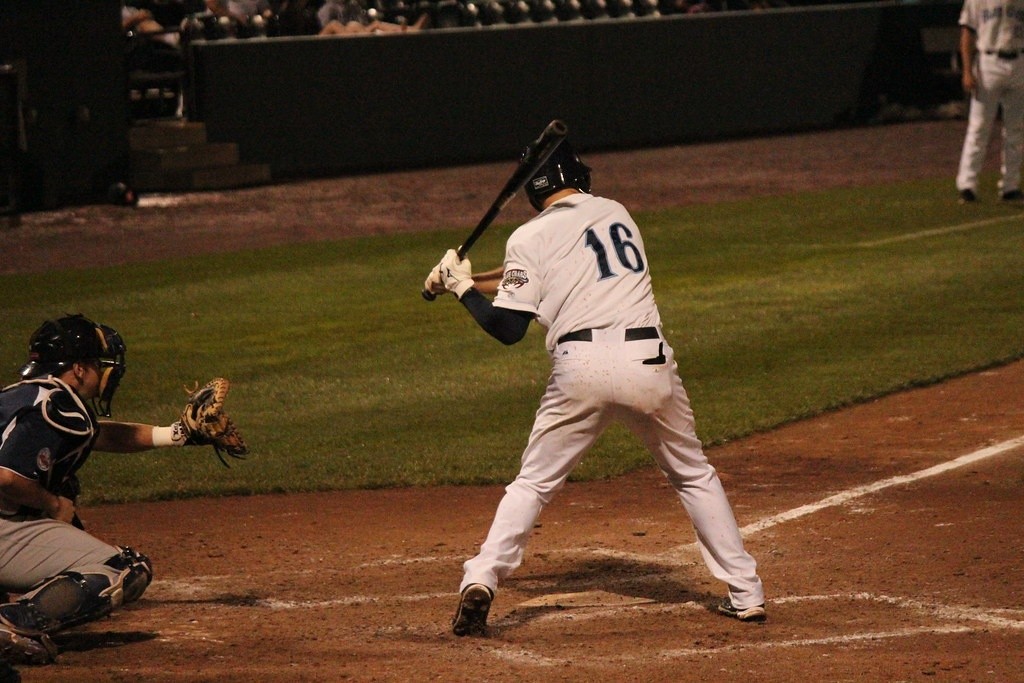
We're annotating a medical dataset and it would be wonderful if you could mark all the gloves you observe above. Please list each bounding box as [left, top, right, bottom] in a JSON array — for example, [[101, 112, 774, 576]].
[[440, 244, 475, 298], [424, 263, 448, 294]]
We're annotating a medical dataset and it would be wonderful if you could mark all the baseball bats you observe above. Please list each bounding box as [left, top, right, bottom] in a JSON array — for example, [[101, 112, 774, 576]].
[[418, 117, 570, 305]]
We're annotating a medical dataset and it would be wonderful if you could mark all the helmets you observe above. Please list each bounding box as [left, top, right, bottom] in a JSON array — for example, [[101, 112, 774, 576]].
[[20, 313, 127, 417], [519, 136, 594, 210]]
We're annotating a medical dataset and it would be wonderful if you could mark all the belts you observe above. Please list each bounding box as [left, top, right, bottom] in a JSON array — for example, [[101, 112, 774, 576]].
[[556, 327, 660, 342], [985, 49, 1024, 60]]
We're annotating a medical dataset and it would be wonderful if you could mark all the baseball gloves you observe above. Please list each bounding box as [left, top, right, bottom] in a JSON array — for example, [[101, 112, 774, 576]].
[[177, 375, 251, 458]]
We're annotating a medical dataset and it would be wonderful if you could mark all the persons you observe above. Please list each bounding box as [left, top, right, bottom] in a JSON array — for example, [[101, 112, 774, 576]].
[[0, 311, 251, 665], [122, 0, 706, 44], [956, 0, 1024, 202], [425, 140, 767, 635]]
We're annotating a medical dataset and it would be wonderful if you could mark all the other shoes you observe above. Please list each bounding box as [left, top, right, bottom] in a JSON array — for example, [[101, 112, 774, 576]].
[[452, 582, 493, 636], [718, 594, 768, 621], [0, 597, 56, 664], [958, 190, 978, 206], [996, 189, 1024, 205]]
